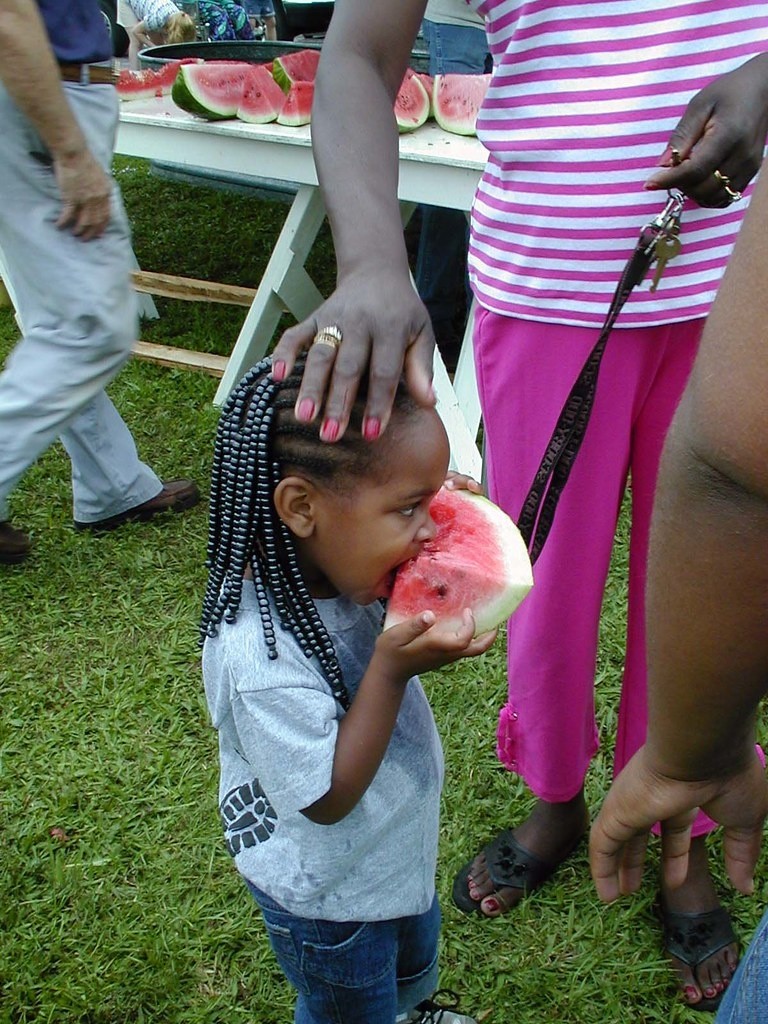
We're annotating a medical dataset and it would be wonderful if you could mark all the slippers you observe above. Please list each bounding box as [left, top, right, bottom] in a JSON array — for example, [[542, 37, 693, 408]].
[[654, 888, 739, 1012], [453, 826, 588, 919]]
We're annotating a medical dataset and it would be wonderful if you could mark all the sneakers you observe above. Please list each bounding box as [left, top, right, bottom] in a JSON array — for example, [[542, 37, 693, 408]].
[[0, 520, 34, 564], [74, 478, 200, 531]]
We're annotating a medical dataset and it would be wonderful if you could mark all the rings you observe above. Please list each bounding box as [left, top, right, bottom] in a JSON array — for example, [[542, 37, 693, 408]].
[[714, 170, 731, 186], [312, 325, 343, 348], [725, 187, 743, 201]]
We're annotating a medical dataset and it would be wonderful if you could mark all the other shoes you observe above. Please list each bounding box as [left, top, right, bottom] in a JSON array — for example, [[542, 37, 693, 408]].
[[396, 989, 477, 1024]]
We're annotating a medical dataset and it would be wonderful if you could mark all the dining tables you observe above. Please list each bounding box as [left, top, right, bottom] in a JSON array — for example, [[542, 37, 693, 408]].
[[113, 93, 490, 483]]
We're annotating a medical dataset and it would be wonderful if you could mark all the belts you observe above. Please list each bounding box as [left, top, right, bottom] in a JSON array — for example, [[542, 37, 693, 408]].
[[58, 56, 120, 84]]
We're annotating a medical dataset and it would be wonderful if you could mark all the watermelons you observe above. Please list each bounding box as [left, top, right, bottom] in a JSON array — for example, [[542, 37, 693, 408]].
[[115, 50, 320, 126], [394, 68, 492, 136], [382, 484, 534, 640]]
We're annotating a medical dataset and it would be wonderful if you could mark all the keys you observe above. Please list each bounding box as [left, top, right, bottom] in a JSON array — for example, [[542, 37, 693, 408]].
[[643, 149, 682, 293]]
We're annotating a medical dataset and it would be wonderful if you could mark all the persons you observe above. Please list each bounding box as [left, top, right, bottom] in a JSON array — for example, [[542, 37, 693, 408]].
[[267, 0, 768, 1013], [242, 0, 277, 41], [200, 353, 500, 1024], [173, 0, 255, 41], [115, 0, 196, 71], [413, 0, 494, 372], [0, 0, 199, 563], [589, 148, 768, 1024]]
